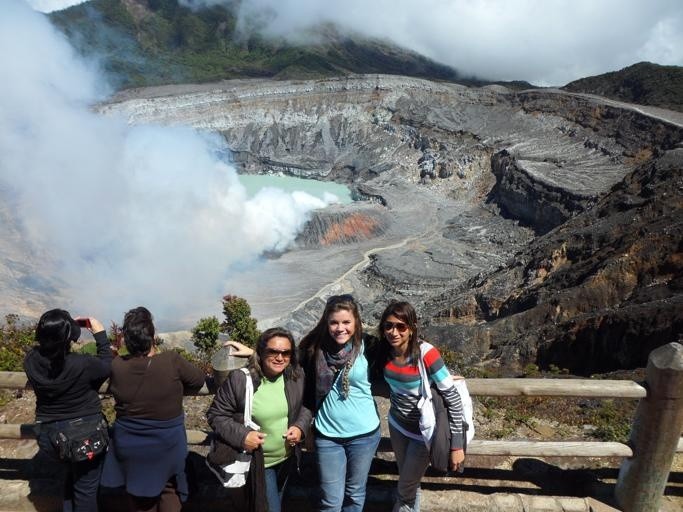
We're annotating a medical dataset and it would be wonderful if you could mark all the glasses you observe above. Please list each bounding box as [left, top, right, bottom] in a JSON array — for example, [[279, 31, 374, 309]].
[[263, 348, 293, 358], [381, 321, 410, 332]]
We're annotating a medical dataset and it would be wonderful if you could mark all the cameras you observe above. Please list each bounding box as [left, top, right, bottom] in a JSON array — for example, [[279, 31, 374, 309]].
[[74, 318, 91, 328]]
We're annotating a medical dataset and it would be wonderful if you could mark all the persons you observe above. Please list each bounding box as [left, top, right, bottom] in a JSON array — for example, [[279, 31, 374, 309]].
[[198, 326, 316, 512], [375, 303, 465, 512], [221, 294, 384, 511], [22, 308, 114, 512], [96, 306, 205, 512]]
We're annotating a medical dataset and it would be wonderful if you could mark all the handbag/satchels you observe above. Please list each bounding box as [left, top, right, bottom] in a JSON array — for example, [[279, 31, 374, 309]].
[[416, 375, 475, 455], [205, 413, 260, 488], [47, 414, 108, 462]]
